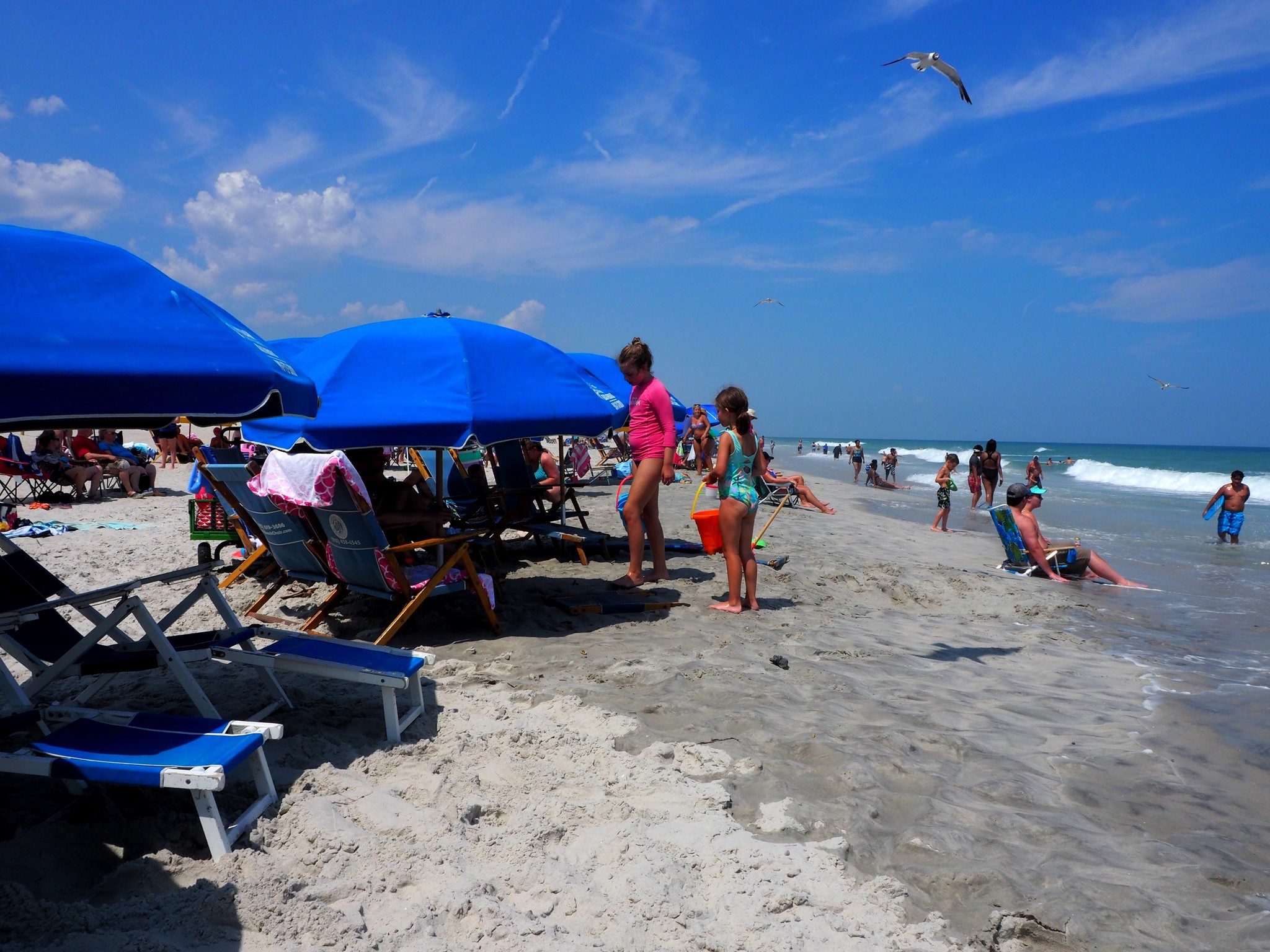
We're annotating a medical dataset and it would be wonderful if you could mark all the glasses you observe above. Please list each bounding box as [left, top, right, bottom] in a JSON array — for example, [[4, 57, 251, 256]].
[[769, 460, 771, 463], [693, 404, 701, 407], [108, 432, 117, 434], [54, 438, 60, 441], [214, 430, 219, 432]]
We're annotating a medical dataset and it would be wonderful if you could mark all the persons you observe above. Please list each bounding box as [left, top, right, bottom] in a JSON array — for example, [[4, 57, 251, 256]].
[[931, 454, 960, 532], [1059, 457, 1077, 464], [71, 428, 154, 499], [761, 450, 837, 515], [864, 459, 911, 489], [1025, 455, 1043, 488], [1204, 471, 1252, 544], [396, 446, 406, 463], [345, 444, 498, 581], [1045, 458, 1053, 465], [467, 463, 488, 494], [54, 430, 73, 455], [157, 417, 180, 469], [1006, 483, 1149, 589], [968, 439, 1004, 510], [97, 428, 167, 498], [682, 387, 775, 612], [518, 441, 568, 508], [210, 427, 229, 449], [882, 448, 905, 483], [176, 424, 201, 454], [611, 337, 677, 589], [797, 439, 853, 461], [33, 430, 107, 503], [402, 466, 437, 501], [848, 440, 865, 481]]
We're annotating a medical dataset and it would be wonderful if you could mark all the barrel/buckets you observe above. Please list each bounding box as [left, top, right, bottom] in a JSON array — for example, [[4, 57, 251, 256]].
[[570, 475, 578, 482], [614, 475, 646, 534], [705, 485, 718, 500], [690, 481, 723, 555]]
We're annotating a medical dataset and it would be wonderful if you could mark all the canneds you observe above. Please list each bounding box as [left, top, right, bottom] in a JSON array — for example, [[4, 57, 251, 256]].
[[1074, 538, 1081, 547]]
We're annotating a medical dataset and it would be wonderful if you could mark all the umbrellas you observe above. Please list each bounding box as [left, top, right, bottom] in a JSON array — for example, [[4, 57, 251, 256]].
[[675, 402, 720, 434], [559, 350, 687, 529], [0, 225, 321, 430], [243, 311, 629, 578]]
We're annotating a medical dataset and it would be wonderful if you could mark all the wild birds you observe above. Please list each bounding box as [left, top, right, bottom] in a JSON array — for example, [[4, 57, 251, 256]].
[[1147, 374, 1191, 391], [752, 297, 785, 309], [878, 50, 975, 108]]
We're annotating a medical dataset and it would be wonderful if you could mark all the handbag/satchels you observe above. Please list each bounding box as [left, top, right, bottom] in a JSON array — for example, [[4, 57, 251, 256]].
[[0, 503, 17, 525], [196, 499, 237, 531]]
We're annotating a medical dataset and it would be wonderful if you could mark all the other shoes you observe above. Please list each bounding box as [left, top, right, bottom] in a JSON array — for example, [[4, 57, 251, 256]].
[[141, 490, 154, 496], [129, 492, 146, 498], [154, 490, 167, 497]]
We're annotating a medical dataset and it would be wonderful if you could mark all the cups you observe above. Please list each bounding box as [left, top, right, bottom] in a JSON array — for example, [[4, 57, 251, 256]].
[[1073, 538, 1080, 544]]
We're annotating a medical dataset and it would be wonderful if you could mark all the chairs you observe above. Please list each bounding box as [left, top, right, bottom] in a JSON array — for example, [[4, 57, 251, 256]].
[[0, 426, 800, 865], [976, 501, 1077, 577]]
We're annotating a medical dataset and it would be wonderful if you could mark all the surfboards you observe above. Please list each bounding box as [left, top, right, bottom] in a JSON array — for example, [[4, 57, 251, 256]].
[[1204, 494, 1225, 520]]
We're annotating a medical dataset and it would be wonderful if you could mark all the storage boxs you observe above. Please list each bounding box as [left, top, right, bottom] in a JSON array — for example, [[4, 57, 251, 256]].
[[188, 499, 241, 540]]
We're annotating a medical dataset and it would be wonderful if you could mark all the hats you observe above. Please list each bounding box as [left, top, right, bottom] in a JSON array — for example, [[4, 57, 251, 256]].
[[1007, 483, 1031, 497], [747, 408, 758, 419], [763, 451, 774, 459], [1030, 485, 1047, 494], [974, 449, 983, 453]]
